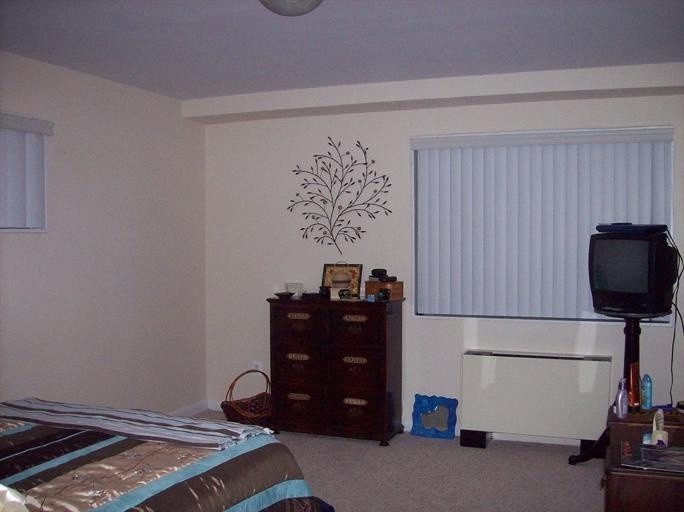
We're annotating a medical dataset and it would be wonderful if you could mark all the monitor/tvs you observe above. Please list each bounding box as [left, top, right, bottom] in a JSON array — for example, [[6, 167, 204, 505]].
[[588, 232, 678, 313]]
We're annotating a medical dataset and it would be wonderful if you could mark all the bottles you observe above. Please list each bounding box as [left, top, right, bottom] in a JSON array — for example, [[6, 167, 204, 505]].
[[338, 289, 351, 299]]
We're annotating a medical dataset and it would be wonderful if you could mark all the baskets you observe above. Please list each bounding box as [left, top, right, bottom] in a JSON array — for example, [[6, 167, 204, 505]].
[[220, 369, 276, 426]]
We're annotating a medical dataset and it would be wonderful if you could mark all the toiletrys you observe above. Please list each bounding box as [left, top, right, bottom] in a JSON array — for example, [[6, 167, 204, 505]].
[[642, 434, 651, 444]]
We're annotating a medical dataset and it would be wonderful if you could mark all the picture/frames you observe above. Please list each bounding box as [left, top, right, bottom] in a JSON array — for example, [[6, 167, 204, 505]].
[[410, 394, 459, 439], [322, 264, 363, 299]]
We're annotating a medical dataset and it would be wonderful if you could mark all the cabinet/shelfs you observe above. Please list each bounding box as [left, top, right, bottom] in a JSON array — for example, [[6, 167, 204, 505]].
[[266, 295, 405, 446], [607, 405, 684, 445], [601, 446, 684, 512]]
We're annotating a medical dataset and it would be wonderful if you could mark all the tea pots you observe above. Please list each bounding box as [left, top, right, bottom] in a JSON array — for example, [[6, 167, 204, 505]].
[[326, 261, 352, 288], [377, 288, 391, 301]]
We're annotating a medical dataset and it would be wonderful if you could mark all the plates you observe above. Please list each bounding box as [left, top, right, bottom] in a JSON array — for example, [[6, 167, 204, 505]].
[[275, 293, 295, 298]]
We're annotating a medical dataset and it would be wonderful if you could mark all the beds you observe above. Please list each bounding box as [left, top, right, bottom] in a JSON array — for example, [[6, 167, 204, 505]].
[[0, 398, 335, 512]]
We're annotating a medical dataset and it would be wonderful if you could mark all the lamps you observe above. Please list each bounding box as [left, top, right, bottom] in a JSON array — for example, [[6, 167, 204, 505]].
[[259, 0, 323, 16]]
